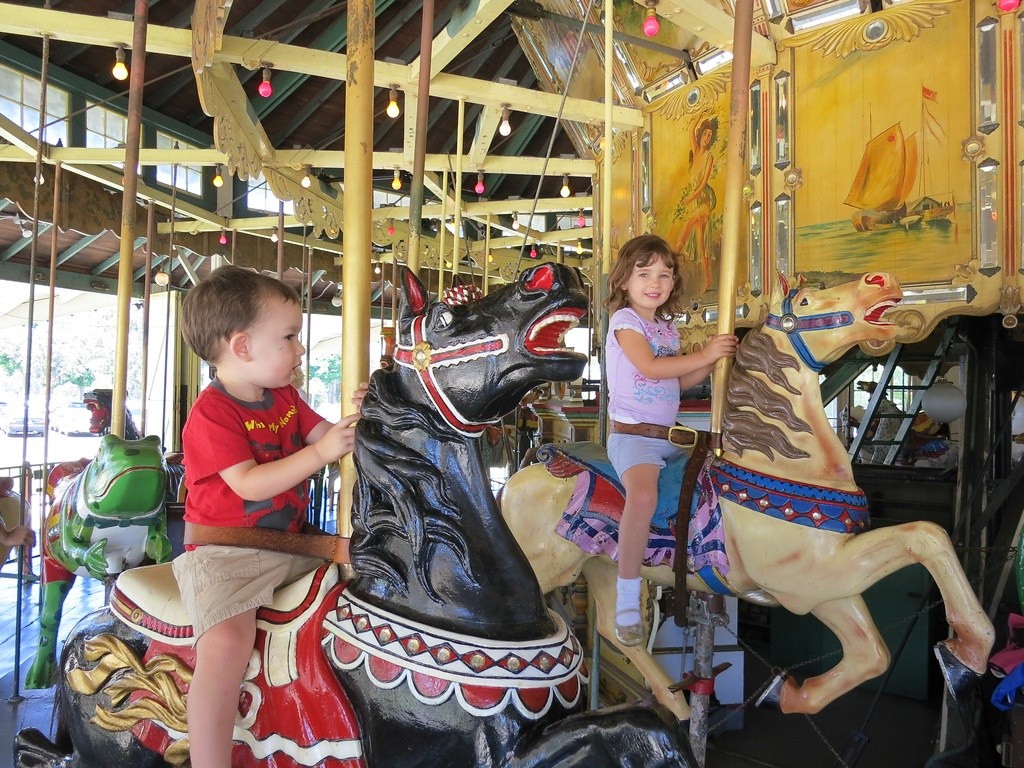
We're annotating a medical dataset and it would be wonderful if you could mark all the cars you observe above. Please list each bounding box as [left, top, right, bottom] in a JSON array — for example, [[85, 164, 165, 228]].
[[49, 402, 100, 437], [0, 402, 46, 437]]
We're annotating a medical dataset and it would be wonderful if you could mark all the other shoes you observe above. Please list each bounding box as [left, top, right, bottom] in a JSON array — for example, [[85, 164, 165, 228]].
[[613, 594, 645, 647]]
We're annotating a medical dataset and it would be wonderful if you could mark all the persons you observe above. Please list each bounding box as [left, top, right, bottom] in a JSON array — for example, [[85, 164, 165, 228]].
[[0, 517, 36, 548], [603, 236, 738, 647], [170, 262, 369, 768]]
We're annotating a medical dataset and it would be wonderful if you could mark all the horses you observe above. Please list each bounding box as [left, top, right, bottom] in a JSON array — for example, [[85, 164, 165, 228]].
[[13, 261, 688, 768], [83, 388, 167, 455], [495, 269, 996, 716]]
